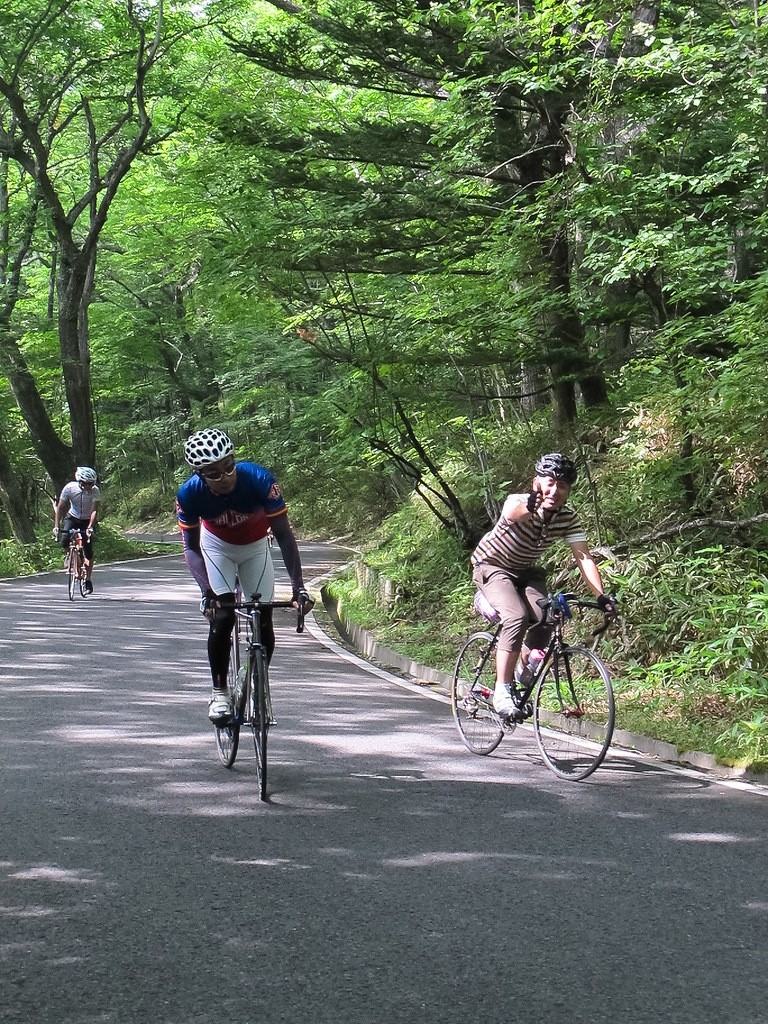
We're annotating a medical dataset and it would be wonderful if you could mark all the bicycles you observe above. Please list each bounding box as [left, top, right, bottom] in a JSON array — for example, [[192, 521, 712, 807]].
[[451, 590, 627, 781], [203, 593, 309, 801], [51, 529, 94, 602]]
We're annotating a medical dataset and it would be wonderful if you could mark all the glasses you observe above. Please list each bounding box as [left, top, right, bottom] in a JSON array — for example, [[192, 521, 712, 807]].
[[198, 457, 235, 481]]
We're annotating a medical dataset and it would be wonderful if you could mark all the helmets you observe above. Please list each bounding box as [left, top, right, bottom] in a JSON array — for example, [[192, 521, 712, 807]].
[[184, 427, 234, 471], [535, 453, 577, 485], [75, 467, 97, 483]]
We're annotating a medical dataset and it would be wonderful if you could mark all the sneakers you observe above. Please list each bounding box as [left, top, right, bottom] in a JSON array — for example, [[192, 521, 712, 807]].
[[209, 686, 232, 721], [491, 688, 520, 717], [64, 558, 69, 568], [85, 580, 93, 594], [516, 665, 534, 686], [251, 689, 276, 723]]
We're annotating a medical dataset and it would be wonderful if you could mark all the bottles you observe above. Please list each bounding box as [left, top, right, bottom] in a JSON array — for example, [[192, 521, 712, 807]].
[[517, 649, 545, 686], [233, 662, 248, 700]]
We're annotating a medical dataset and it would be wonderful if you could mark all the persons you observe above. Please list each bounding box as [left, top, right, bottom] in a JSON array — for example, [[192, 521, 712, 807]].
[[173, 428, 315, 720], [53, 467, 102, 594], [469, 453, 616, 722]]
